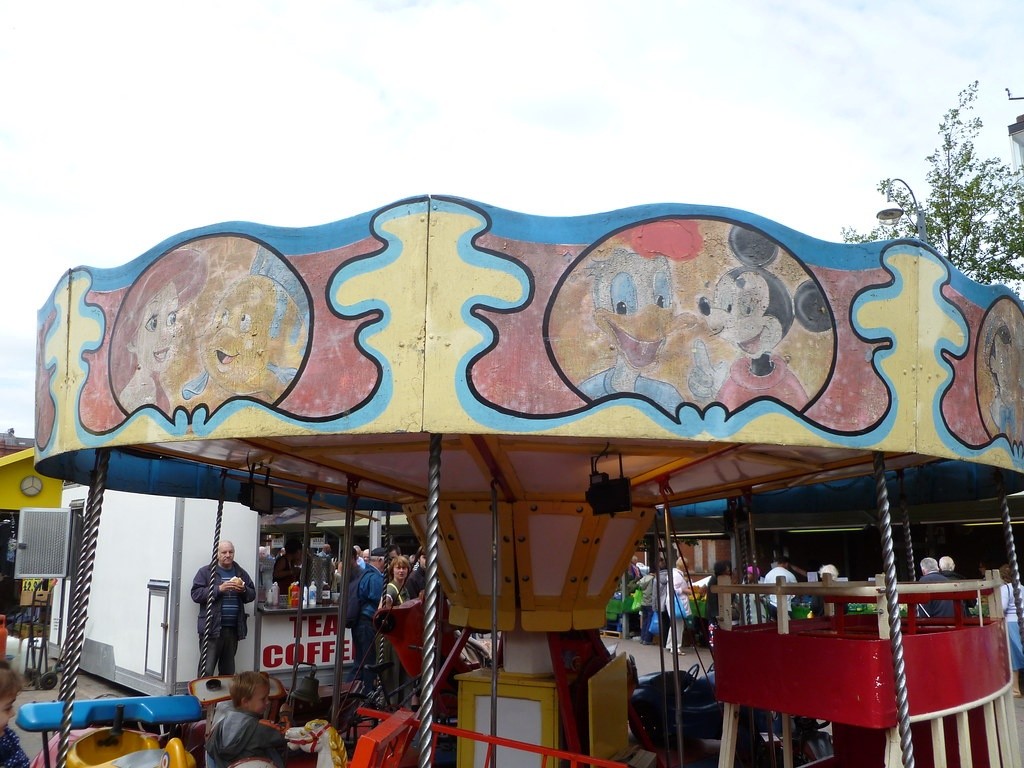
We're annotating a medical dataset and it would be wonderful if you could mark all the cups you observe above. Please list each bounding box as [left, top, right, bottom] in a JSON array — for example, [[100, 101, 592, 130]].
[[331, 593, 340, 604]]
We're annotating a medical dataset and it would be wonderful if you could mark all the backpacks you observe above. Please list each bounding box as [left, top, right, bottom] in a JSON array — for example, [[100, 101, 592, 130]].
[[346, 570, 374, 628]]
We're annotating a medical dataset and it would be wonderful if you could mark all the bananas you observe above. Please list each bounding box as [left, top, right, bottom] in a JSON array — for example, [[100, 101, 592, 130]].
[[688, 593, 707, 601]]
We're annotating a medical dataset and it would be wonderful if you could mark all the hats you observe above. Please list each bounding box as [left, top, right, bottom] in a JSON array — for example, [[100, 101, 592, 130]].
[[371, 547, 392, 561]]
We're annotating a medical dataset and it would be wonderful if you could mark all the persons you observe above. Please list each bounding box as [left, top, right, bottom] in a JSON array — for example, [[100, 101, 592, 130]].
[[0, 659, 30, 768], [605, 555, 1024, 698], [203, 671, 291, 768], [259, 537, 476, 713], [191, 540, 256, 679]]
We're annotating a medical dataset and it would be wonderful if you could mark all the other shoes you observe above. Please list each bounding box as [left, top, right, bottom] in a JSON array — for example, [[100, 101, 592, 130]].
[[670, 648, 686, 655], [640, 640, 658, 645]]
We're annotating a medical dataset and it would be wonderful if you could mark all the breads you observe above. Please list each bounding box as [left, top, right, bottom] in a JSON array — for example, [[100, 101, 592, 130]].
[[231, 576, 243, 590]]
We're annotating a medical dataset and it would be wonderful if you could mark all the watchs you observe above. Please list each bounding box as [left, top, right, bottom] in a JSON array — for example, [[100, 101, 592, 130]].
[[243, 589, 246, 592]]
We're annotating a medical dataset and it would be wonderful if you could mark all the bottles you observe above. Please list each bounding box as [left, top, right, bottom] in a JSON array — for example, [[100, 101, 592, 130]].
[[291, 581, 299, 607], [322, 582, 331, 607], [267, 589, 272, 605], [302, 586, 308, 606], [288, 582, 295, 604], [272, 582, 281, 606], [309, 581, 317, 605]]
